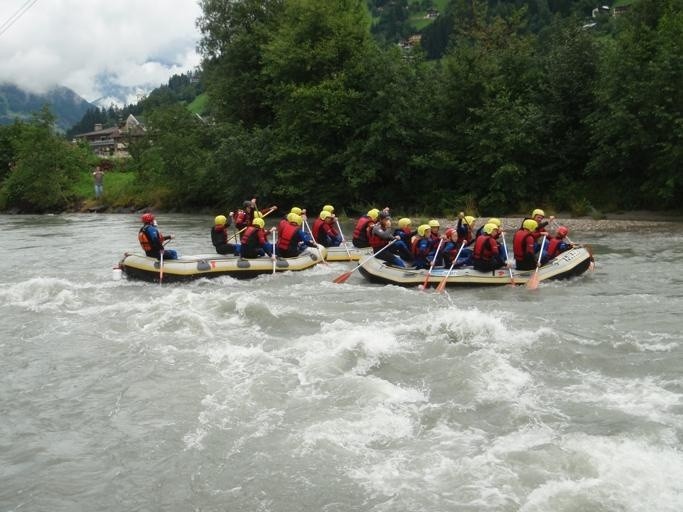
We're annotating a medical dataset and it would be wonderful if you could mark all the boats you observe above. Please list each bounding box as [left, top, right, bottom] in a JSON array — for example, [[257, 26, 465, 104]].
[[320, 241, 373, 264], [120, 240, 325, 282], [356, 244, 595, 289]]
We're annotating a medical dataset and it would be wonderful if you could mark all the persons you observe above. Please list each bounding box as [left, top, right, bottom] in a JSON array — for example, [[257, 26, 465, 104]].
[[138, 213, 178, 260], [352, 206, 512, 271], [92, 166, 104, 199], [212, 200, 342, 257], [512, 209, 571, 270]]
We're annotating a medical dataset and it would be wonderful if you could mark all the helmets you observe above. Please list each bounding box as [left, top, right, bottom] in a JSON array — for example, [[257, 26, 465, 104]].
[[483, 218, 501, 234], [380, 211, 390, 221], [446, 228, 457, 238], [252, 211, 265, 229], [463, 216, 476, 226], [532, 209, 545, 219], [558, 226, 568, 238], [367, 209, 380, 221], [320, 205, 334, 221], [241, 201, 251, 210], [523, 220, 538, 233], [215, 215, 227, 226], [399, 218, 411, 229], [287, 207, 302, 226], [418, 224, 430, 237], [429, 220, 440, 230], [142, 213, 155, 222]]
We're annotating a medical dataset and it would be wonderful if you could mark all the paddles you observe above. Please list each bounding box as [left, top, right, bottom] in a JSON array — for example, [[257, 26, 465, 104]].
[[422, 239, 444, 291], [501, 231, 517, 288], [436, 240, 467, 293], [527, 234, 546, 289], [552, 218, 594, 271], [331, 239, 398, 284]]
